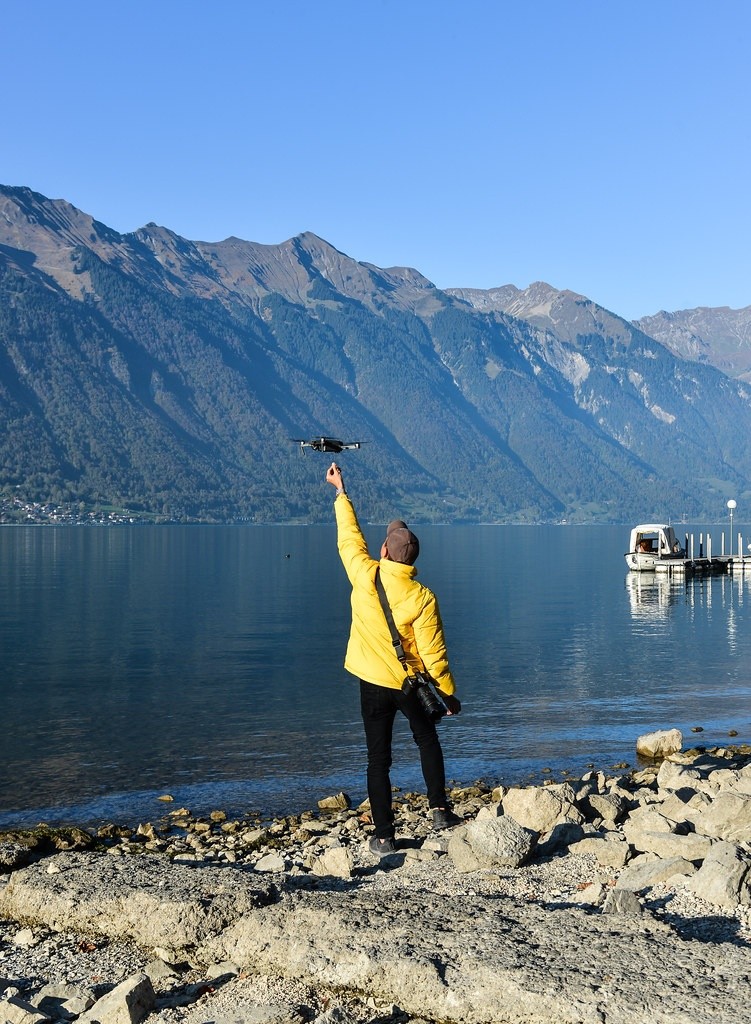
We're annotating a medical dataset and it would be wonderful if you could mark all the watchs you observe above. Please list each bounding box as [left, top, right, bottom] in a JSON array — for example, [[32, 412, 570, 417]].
[[335, 487, 345, 496]]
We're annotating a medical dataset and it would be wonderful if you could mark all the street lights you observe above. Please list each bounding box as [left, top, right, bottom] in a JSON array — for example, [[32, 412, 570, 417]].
[[727, 499, 736, 555]]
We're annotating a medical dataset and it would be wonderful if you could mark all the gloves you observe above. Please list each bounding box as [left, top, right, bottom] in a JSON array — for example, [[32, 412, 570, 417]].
[[442, 696, 462, 715]]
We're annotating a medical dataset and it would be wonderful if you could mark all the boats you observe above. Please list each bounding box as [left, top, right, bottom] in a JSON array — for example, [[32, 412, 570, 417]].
[[624, 523, 685, 569], [626, 570, 689, 623]]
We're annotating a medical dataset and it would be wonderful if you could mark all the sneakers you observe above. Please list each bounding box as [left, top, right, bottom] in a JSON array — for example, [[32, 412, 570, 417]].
[[368, 834, 396, 856], [431, 807, 464, 831]]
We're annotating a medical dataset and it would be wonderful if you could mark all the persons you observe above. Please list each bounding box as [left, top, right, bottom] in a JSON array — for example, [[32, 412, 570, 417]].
[[321, 462, 463, 856], [637, 539, 650, 554]]
